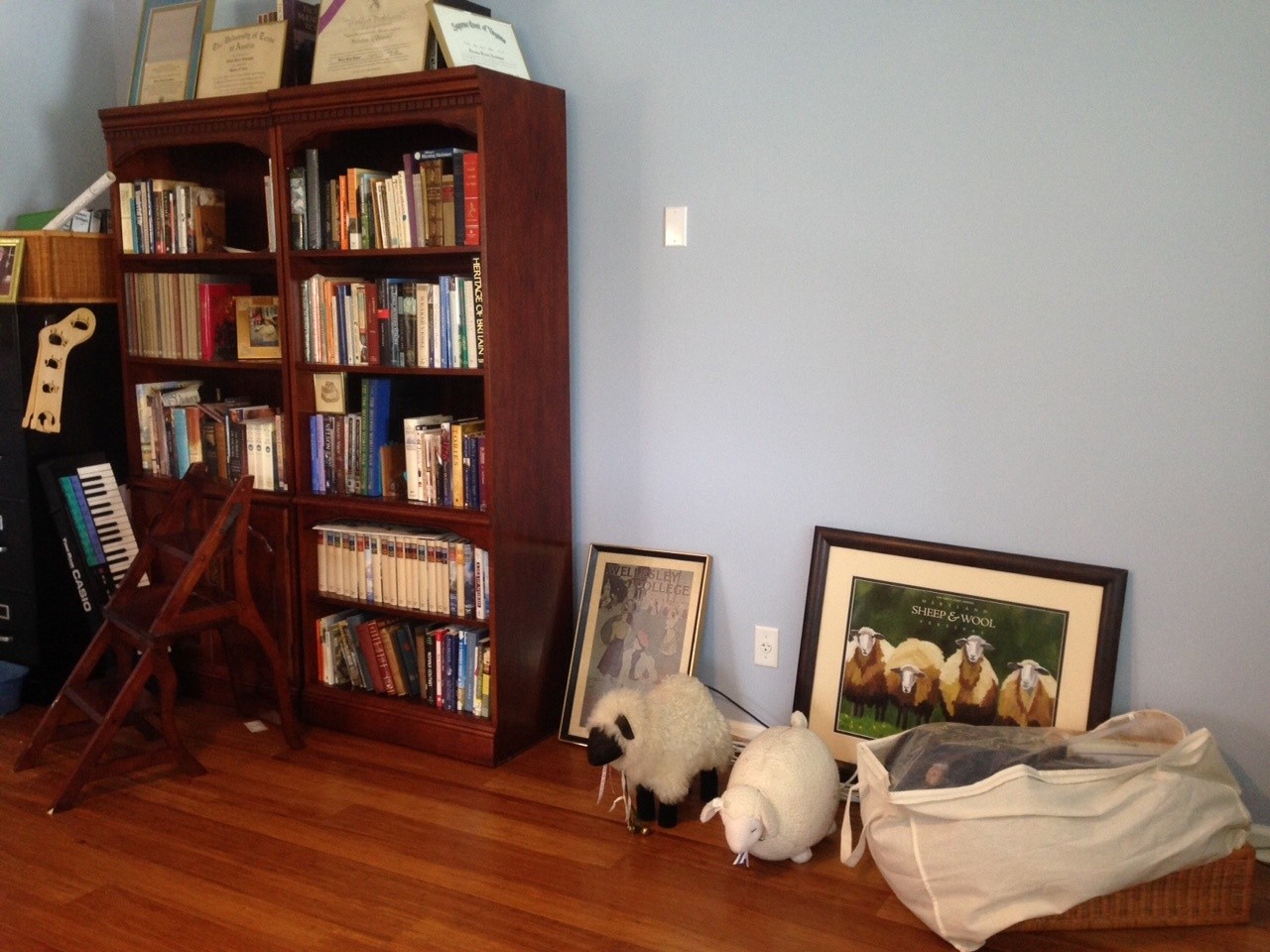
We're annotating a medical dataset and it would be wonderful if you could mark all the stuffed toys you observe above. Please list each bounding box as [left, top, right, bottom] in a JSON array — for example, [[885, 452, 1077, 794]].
[[698, 710, 843, 866], [583, 672, 733, 828]]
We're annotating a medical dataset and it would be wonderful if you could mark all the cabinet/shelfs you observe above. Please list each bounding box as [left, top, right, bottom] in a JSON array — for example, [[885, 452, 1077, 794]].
[[96, 88, 304, 715], [270, 63, 578, 770]]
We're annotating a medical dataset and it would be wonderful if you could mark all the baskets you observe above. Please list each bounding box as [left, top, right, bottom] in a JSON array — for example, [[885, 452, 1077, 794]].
[[1000, 843, 1254, 928]]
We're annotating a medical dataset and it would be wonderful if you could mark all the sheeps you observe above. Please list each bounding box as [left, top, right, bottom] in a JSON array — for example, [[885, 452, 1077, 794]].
[[700, 711, 843, 869]]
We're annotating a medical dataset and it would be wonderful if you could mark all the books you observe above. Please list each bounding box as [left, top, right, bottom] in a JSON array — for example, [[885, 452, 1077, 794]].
[[99, 146, 491, 725]]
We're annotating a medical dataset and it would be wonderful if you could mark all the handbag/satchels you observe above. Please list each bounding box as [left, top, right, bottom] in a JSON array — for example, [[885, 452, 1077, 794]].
[[839, 708, 1254, 952]]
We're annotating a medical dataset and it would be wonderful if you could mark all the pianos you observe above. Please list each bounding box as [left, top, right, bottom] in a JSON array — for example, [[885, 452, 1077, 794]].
[[36, 441, 157, 678]]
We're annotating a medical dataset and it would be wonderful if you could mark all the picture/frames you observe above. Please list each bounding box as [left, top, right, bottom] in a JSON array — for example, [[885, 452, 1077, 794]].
[[236, 295, 282, 359], [790, 523, 1126, 792], [1, 236, 26, 303], [556, 538, 708, 747]]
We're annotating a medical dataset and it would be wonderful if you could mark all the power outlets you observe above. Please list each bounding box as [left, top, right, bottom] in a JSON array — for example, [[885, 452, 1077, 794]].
[[754, 625, 778, 667]]
